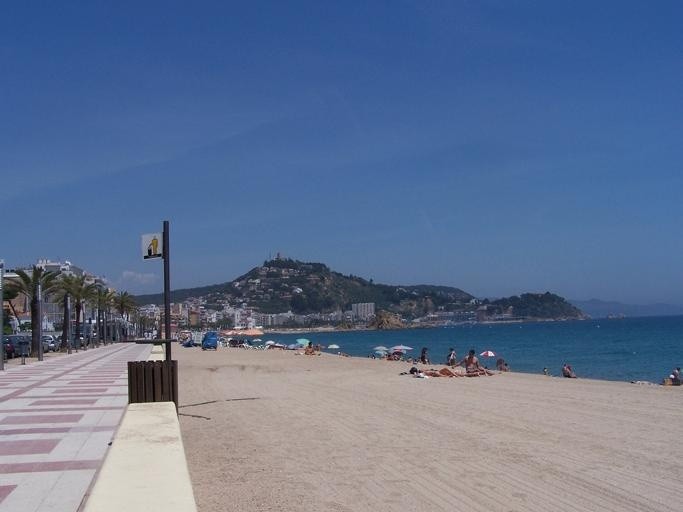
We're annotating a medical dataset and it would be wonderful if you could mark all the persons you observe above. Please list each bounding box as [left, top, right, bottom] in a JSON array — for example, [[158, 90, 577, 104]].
[[419, 346, 432, 364], [303, 341, 315, 355], [561, 363, 570, 376], [672, 367, 680, 379], [452, 349, 488, 375], [446, 346, 457, 366]]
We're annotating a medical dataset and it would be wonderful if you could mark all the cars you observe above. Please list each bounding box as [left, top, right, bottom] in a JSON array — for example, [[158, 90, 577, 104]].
[[2, 331, 97, 360]]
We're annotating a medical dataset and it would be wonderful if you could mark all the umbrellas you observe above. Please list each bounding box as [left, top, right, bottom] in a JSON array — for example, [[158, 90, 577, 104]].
[[479, 350, 496, 360], [327, 343, 339, 349], [296, 338, 312, 348], [373, 343, 414, 355]]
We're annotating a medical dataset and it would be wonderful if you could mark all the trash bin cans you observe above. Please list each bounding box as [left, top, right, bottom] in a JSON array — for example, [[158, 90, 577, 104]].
[[127, 360, 178, 418]]
